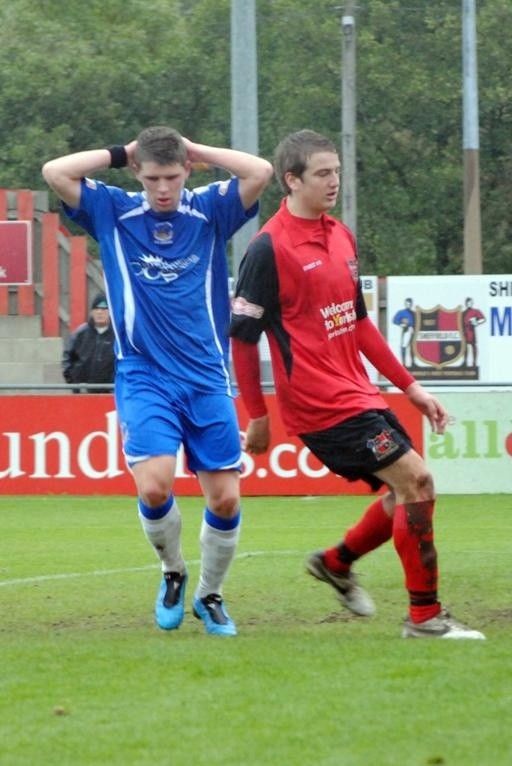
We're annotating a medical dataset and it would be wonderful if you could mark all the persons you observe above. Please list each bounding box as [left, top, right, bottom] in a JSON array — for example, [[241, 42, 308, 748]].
[[37, 125, 273, 634], [60, 293, 116, 393], [224, 129, 483, 642], [460, 297, 487, 367], [391, 296, 418, 367]]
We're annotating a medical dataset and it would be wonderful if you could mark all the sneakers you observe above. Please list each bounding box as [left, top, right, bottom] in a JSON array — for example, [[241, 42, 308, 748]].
[[192, 593, 235, 636], [402, 610, 485, 640], [305, 551, 375, 616], [154, 571, 187, 629]]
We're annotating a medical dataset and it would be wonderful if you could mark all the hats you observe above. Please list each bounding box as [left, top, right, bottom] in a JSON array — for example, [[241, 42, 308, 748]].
[[92, 293, 107, 310]]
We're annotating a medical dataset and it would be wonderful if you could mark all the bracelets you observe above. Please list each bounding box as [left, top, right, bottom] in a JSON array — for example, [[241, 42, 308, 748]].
[[106, 144, 127, 169]]
